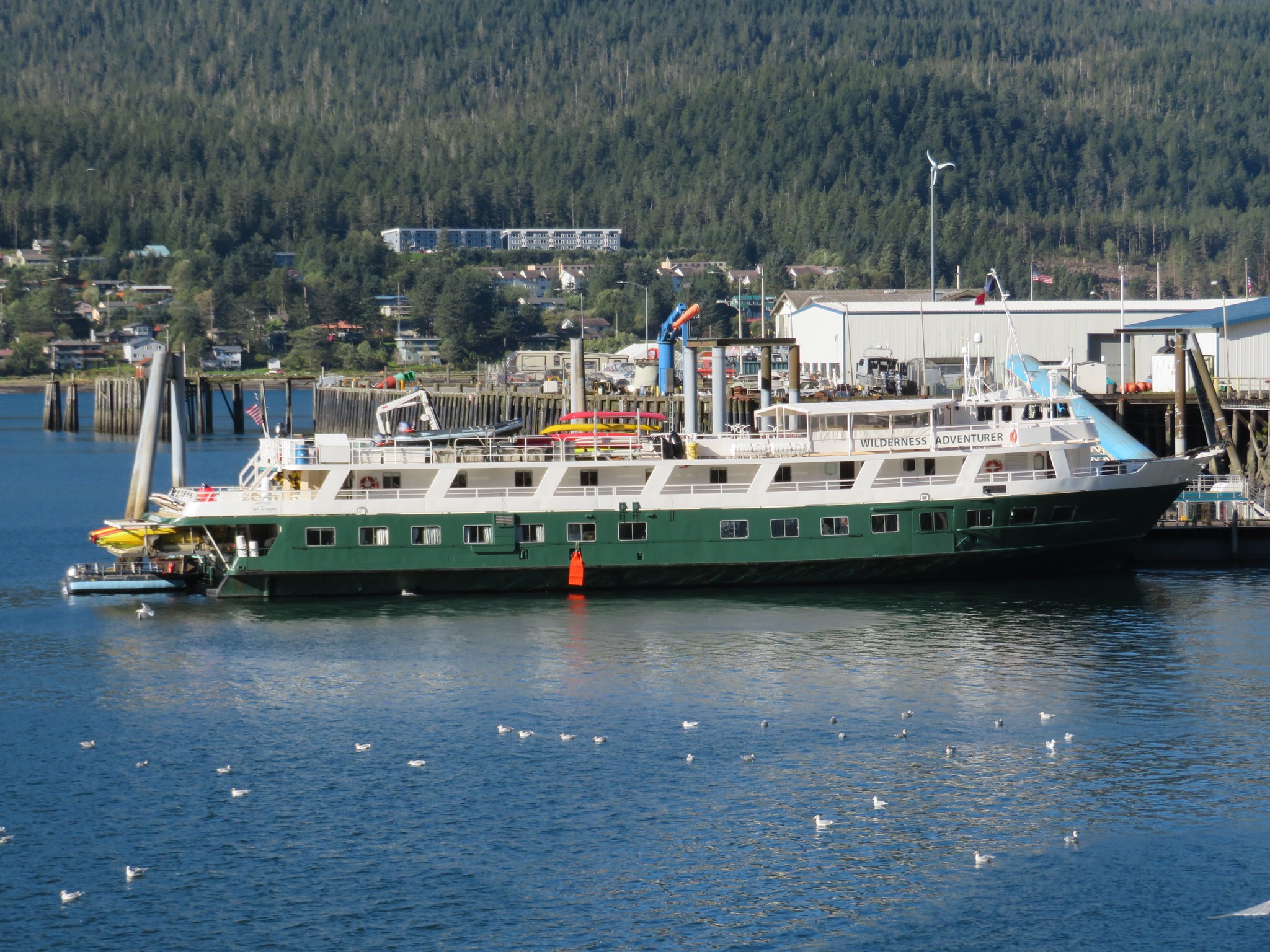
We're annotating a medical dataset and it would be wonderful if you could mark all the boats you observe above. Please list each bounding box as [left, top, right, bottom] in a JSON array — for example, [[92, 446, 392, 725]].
[[115, 320, 1211, 600], [60, 559, 193, 595]]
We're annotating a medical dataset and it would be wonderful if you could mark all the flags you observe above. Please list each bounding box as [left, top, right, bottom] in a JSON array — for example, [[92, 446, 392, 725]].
[[983, 277, 995, 293], [975, 293, 985, 305], [245, 402, 264, 425], [1033, 271, 1053, 285], [1248, 274, 1252, 296]]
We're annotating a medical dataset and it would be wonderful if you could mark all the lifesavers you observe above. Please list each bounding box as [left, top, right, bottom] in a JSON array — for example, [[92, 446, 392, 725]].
[[360, 476, 376, 489], [404, 428, 414, 433], [376, 443, 385, 446], [985, 460, 999, 472], [1010, 429, 1017, 443]]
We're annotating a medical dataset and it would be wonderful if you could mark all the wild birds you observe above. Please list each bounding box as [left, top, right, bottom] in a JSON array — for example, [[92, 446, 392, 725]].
[[135, 760, 149, 767], [59, 889, 86, 901], [1064, 732, 1075, 740], [77, 740, 98, 749], [231, 787, 253, 796], [1045, 739, 1056, 749], [871, 795, 889, 807], [400, 588, 419, 597], [994, 718, 1004, 725], [496, 725, 536, 738], [813, 814, 835, 827], [972, 850, 997, 862], [686, 753, 696, 761], [592, 734, 609, 743], [558, 732, 579, 741], [354, 742, 373, 750], [829, 716, 837, 723], [681, 720, 701, 727], [1038, 712, 1056, 719], [838, 732, 847, 738], [739, 753, 756, 760], [1206, 899, 1270, 920], [0, 825, 19, 844], [407, 759, 431, 767], [1063, 830, 1080, 842], [900, 710, 914, 717], [760, 720, 769, 727], [946, 745, 958, 753], [216, 765, 233, 773], [893, 728, 908, 738], [134, 602, 155, 621], [125, 865, 150, 877]]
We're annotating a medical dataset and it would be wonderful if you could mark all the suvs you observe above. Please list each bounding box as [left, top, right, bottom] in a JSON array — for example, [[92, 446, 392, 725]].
[[546, 369, 570, 384]]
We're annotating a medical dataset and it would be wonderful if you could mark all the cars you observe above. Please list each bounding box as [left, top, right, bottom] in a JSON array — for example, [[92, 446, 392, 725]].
[[589, 361, 636, 388]]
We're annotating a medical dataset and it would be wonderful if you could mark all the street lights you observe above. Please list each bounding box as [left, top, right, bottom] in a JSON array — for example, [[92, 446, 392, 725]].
[[617, 281, 648, 361], [706, 271, 743, 377], [925, 149, 956, 301], [554, 288, 584, 339]]
